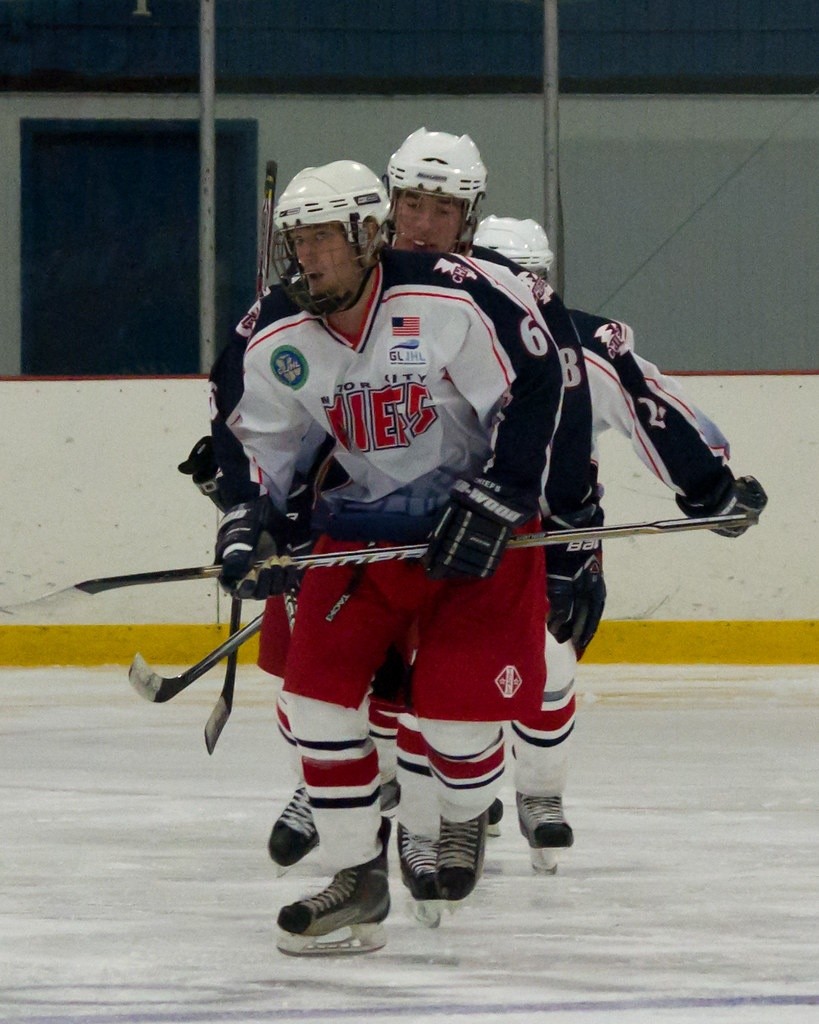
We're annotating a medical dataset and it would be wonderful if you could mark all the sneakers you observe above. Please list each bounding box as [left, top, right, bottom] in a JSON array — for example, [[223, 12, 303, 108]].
[[379, 778, 403, 820], [424, 796, 490, 926], [394, 816, 452, 931], [267, 772, 325, 878], [514, 783, 574, 877], [276, 852, 391, 958]]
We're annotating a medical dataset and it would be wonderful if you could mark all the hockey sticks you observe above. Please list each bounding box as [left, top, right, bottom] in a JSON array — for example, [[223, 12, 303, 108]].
[[129, 608, 266, 703], [203, 161, 277, 754], [1, 511, 759, 620]]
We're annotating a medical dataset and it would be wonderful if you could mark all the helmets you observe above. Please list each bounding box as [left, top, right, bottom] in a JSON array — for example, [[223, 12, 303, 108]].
[[386, 122, 488, 269], [466, 206, 555, 286], [265, 154, 401, 316]]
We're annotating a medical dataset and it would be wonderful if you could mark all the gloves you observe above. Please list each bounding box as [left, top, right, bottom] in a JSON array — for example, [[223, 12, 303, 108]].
[[409, 475, 543, 585], [547, 505, 608, 658], [676, 472, 767, 537], [210, 492, 301, 599]]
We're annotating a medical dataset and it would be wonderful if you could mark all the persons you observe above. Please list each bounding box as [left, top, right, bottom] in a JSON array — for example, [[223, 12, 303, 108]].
[[372, 211, 766, 887], [186, 124, 594, 924], [222, 162, 566, 956]]
